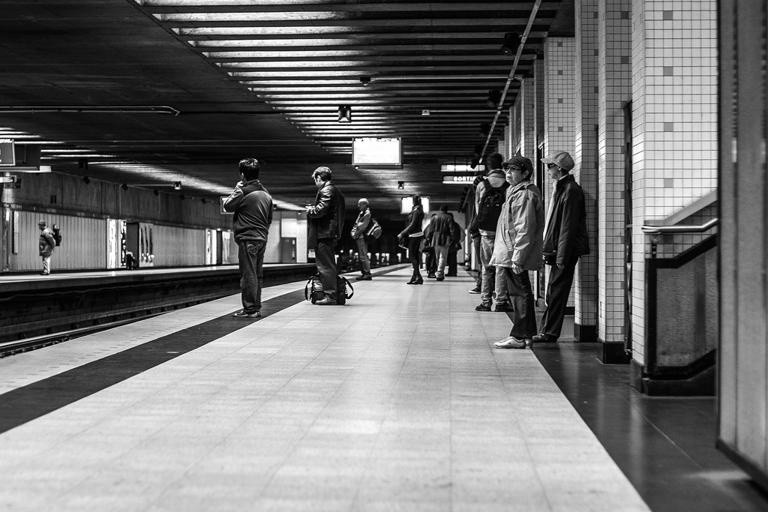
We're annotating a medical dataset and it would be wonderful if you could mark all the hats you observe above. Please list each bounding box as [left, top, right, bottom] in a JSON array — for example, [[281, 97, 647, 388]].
[[540, 152, 574, 170], [502, 156, 534, 175]]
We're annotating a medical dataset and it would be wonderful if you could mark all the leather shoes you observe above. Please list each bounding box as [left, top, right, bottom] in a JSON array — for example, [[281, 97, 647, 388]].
[[406, 277, 423, 284]]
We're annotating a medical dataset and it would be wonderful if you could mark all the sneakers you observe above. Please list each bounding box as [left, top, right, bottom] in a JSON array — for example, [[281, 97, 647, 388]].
[[428, 274, 457, 281], [495, 304, 513, 312], [525, 339, 532, 345], [476, 305, 491, 310], [469, 287, 481, 294], [315, 298, 335, 304], [233, 310, 260, 317], [494, 336, 526, 349], [356, 276, 372, 280], [533, 335, 556, 343]]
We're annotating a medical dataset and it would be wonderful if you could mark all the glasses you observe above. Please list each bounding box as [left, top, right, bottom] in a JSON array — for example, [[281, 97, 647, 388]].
[[548, 164, 556, 168]]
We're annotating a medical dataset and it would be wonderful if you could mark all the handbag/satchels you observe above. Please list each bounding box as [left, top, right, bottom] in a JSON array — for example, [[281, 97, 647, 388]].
[[311, 277, 346, 305], [365, 219, 382, 240], [408, 229, 424, 241], [399, 236, 408, 249]]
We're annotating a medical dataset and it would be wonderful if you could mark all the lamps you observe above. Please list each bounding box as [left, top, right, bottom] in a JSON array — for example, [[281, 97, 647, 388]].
[[397, 181, 405, 190], [337, 106, 352, 123]]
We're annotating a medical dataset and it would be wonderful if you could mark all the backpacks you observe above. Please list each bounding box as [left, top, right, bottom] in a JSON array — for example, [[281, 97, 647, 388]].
[[477, 179, 510, 231]]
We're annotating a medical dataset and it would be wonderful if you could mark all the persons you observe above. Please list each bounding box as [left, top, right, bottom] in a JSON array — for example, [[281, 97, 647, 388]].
[[396, 150, 591, 349], [303, 166, 346, 305], [224, 157, 273, 318], [126, 249, 134, 269], [37, 219, 56, 275], [350, 198, 373, 280]]
